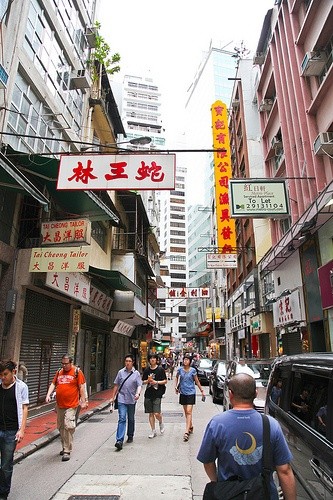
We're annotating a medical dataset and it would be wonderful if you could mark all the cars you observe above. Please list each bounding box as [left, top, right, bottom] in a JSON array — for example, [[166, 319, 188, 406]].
[[190, 359, 229, 403]]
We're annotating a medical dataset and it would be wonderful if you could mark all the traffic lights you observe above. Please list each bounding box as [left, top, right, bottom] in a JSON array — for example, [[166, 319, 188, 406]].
[[157, 347, 162, 350]]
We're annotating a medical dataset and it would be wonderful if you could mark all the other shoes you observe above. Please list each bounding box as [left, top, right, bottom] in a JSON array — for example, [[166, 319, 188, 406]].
[[115, 441, 122, 449], [184, 427, 193, 441], [149, 431, 157, 438], [127, 438, 133, 443], [168, 378, 170, 380], [160, 424, 164, 433], [60, 449, 70, 461], [287, 439, 290, 443], [293, 442, 301, 451]]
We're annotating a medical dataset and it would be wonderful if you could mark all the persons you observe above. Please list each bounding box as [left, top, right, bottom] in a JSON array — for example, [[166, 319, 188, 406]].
[[108, 354, 142, 449], [140, 353, 168, 438], [45, 354, 90, 461], [311, 403, 329, 479], [267, 381, 283, 419], [286, 387, 310, 453], [174, 356, 206, 440], [0, 359, 29, 500], [195, 373, 297, 500], [160, 349, 218, 381]]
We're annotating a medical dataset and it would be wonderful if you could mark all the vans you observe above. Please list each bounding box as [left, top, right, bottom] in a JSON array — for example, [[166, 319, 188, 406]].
[[264, 350, 333, 499]]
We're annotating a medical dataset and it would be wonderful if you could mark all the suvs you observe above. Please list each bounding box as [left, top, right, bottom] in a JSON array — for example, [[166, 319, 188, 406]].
[[223, 356, 274, 414]]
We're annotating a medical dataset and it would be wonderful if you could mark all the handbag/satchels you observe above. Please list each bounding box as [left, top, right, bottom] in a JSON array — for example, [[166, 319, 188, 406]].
[[114, 393, 118, 409], [203, 473, 270, 500]]
